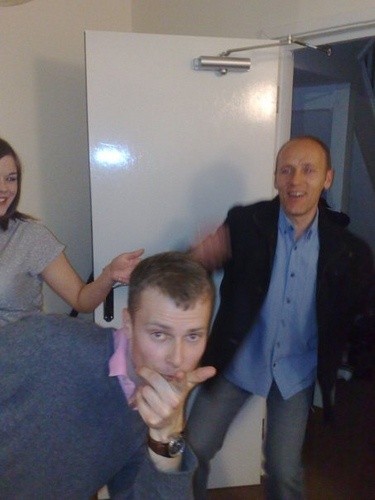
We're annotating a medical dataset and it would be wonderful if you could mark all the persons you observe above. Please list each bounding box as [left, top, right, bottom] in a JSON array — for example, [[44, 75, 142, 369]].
[[0, 137, 144, 329], [0, 252, 216, 500], [188, 135, 375, 500]]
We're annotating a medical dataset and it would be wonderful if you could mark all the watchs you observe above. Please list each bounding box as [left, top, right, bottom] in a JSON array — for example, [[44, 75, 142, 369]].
[[147, 428, 189, 458]]
